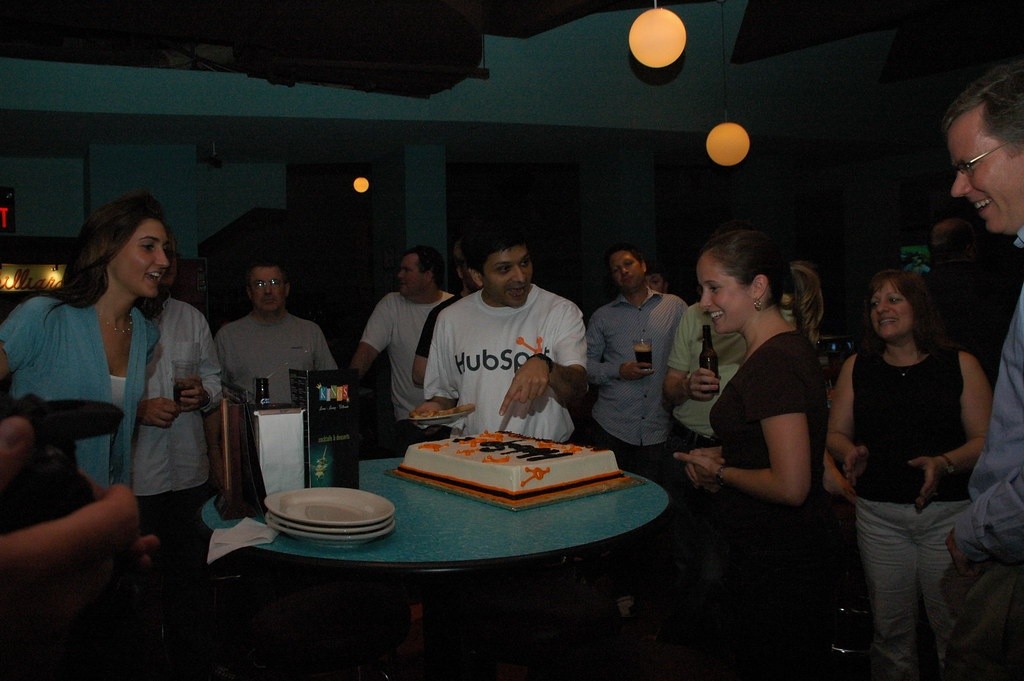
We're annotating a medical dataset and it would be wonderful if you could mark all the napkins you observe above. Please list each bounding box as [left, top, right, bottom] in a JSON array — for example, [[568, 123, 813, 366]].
[[206, 516, 282, 565]]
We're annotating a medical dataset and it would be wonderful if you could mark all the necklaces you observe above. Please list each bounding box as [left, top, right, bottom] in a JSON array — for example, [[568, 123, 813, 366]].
[[897, 361, 915, 377], [98, 311, 134, 335]]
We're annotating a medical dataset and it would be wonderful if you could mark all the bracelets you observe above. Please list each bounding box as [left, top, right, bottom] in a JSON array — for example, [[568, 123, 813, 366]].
[[527, 353, 553, 374], [716, 464, 725, 487]]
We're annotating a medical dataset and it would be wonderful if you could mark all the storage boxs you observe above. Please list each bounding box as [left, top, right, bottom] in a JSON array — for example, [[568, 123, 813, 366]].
[[253, 367, 362, 498]]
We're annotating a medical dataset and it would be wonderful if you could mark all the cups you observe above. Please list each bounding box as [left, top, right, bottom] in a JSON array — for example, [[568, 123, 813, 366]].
[[255, 377, 270, 410], [171, 358, 196, 407], [633, 338, 653, 371]]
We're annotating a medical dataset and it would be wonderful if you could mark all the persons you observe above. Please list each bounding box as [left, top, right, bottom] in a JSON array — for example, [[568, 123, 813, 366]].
[[823, 271, 993, 681], [1, 193, 173, 681], [126, 228, 241, 681], [211, 233, 800, 647], [1, 346, 161, 649], [943, 61, 1023, 680], [668, 229, 834, 681], [925, 219, 1001, 383]]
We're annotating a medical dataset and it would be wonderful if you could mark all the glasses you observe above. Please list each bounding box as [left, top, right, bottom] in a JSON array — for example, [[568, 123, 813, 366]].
[[250, 278, 289, 289], [946, 138, 1022, 181]]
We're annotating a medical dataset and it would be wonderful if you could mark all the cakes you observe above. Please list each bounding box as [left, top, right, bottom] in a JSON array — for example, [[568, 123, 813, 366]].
[[397, 431, 624, 503]]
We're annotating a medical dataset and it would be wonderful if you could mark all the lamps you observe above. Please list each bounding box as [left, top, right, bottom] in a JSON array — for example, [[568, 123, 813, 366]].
[[628, 0, 687, 68], [706, 0, 750, 166]]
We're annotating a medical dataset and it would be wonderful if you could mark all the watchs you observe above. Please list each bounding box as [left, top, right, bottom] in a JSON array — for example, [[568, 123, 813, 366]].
[[941, 454, 955, 473]]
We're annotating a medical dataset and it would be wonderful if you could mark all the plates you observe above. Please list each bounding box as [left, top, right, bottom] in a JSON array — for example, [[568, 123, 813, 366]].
[[407, 412, 464, 425], [263, 486, 396, 548]]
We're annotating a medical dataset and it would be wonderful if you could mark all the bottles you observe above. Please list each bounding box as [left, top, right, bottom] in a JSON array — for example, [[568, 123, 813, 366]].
[[699, 324, 720, 395]]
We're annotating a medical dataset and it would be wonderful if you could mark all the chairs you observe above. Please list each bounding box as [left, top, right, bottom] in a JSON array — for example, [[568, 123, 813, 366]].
[[200, 450, 670, 681]]
